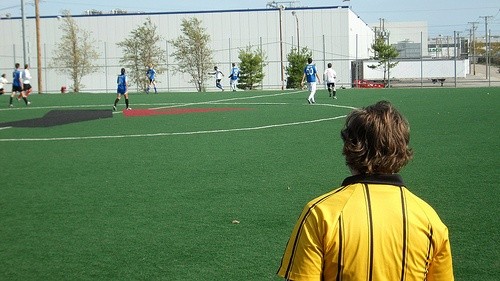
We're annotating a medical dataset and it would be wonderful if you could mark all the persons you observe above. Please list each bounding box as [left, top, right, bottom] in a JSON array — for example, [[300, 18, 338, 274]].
[[276, 100, 457, 281], [16, 64, 32, 104], [207, 66, 225, 92], [226, 63, 241, 92], [301, 58, 321, 104], [323, 63, 338, 99], [0, 73, 9, 96], [144, 63, 158, 94], [9, 63, 32, 108], [113, 67, 132, 112]]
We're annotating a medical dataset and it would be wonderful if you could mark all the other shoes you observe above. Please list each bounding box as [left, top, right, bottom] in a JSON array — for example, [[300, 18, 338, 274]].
[[112, 106, 116, 111], [312, 101, 316, 104], [333, 96, 338, 99], [126, 107, 131, 110], [307, 99, 311, 104]]
